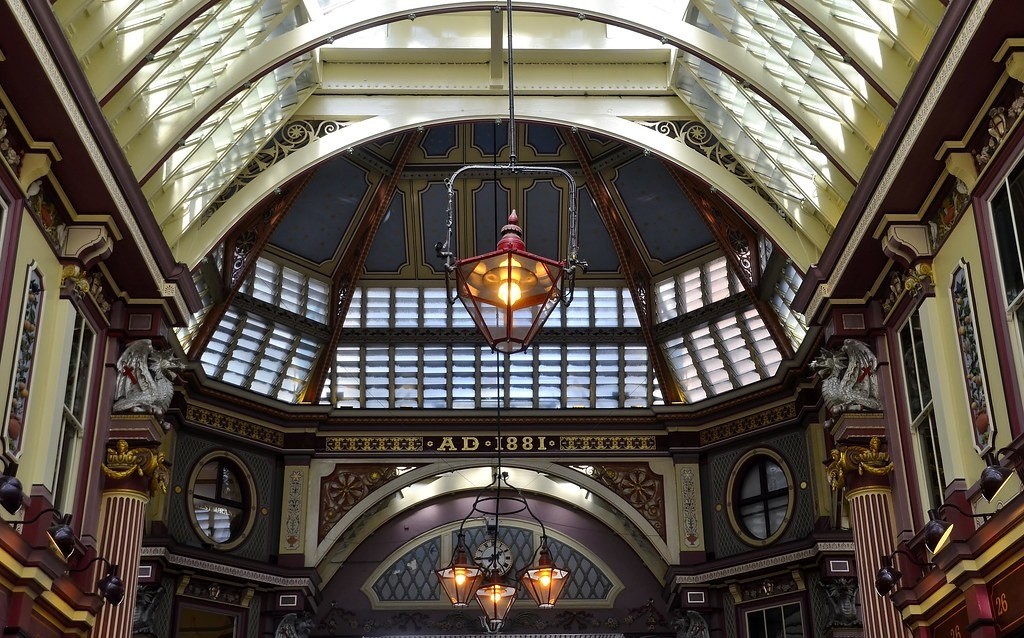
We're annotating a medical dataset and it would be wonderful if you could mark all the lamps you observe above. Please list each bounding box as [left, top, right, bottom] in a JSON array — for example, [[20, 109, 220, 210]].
[[874, 549, 935, 598], [4, 507, 76, 562], [433, 0, 589, 354], [923, 503, 997, 555], [979, 446, 1023, 504], [433, 120, 573, 637], [67, 555, 126, 607], [0, 461, 24, 516]]
